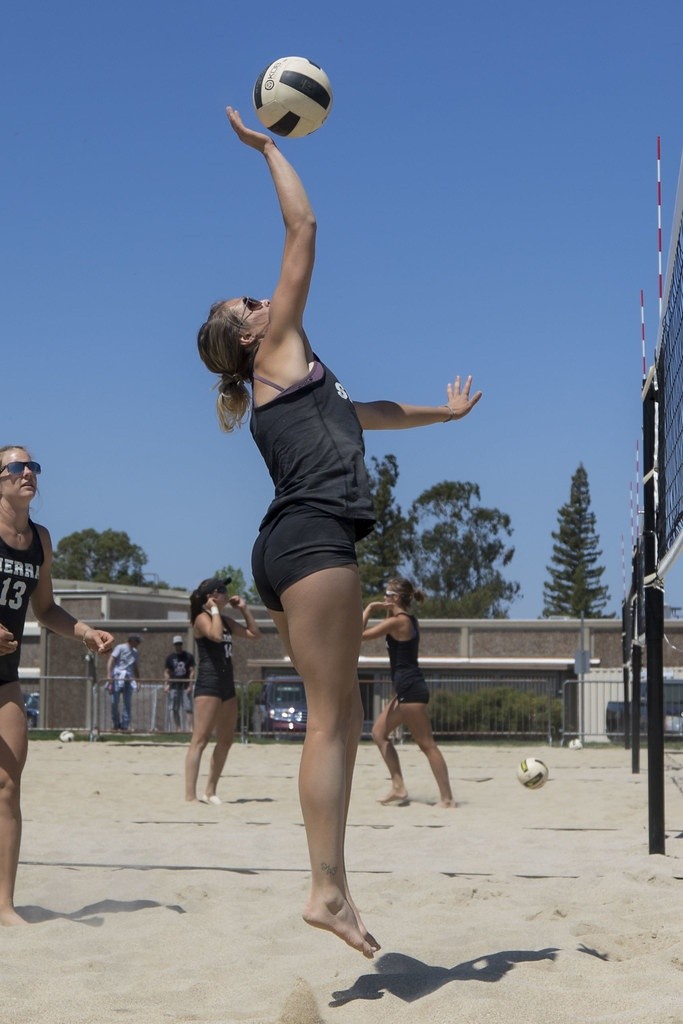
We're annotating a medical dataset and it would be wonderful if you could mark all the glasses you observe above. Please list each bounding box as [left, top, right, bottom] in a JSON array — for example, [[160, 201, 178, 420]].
[[213, 585, 229, 594], [239, 296, 262, 320], [0, 460, 43, 476], [385, 590, 400, 597]]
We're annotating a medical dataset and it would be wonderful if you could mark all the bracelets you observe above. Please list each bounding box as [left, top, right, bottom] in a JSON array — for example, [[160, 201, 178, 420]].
[[441, 405, 455, 423], [82, 629, 96, 661], [211, 607, 220, 615]]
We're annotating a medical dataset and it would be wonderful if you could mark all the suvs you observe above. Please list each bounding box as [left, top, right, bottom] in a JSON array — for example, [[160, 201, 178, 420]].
[[255, 675, 308, 740]]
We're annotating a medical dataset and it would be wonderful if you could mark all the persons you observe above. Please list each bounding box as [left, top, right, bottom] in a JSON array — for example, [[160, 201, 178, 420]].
[[199, 108, 483, 959], [0, 444, 116, 929], [164, 635, 196, 732], [362, 578, 453, 809], [105, 633, 144, 732], [184, 578, 260, 804]]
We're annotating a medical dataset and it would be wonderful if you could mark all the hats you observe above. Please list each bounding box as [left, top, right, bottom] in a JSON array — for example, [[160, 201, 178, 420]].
[[172, 635, 184, 645], [128, 632, 145, 643], [204, 577, 233, 597]]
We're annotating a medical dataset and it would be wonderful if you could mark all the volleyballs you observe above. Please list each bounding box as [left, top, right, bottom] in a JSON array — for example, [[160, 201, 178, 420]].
[[568, 738, 583, 750], [250, 53, 335, 140], [515, 757, 550, 791], [58, 730, 75, 743]]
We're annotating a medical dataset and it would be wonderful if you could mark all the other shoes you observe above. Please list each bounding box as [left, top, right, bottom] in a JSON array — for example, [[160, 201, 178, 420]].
[[120, 726, 135, 733], [202, 794, 222, 805]]
